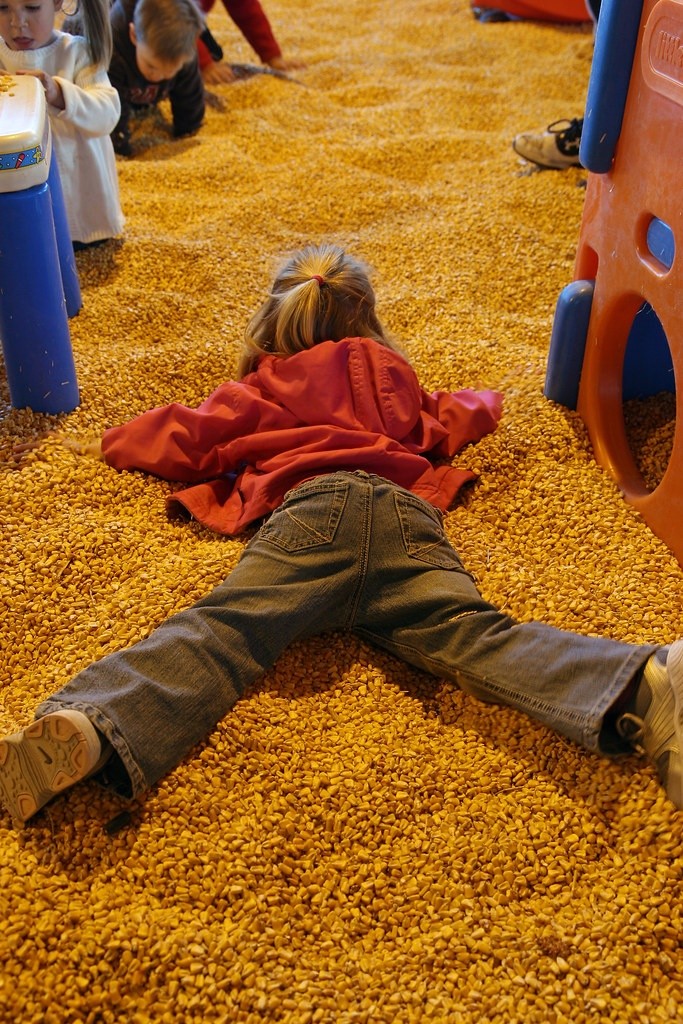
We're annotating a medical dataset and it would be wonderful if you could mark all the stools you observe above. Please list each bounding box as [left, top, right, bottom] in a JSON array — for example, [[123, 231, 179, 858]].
[[0, 76, 83, 417]]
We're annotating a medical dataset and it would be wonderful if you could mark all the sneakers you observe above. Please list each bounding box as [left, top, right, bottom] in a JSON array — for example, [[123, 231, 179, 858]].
[[513, 116, 584, 170], [0, 709, 110, 822], [617, 640, 683, 812]]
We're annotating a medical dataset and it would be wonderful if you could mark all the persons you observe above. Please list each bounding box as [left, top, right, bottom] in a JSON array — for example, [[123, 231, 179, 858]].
[[0, 243, 683, 816], [510, 1, 601, 172], [0, 0, 310, 252]]
